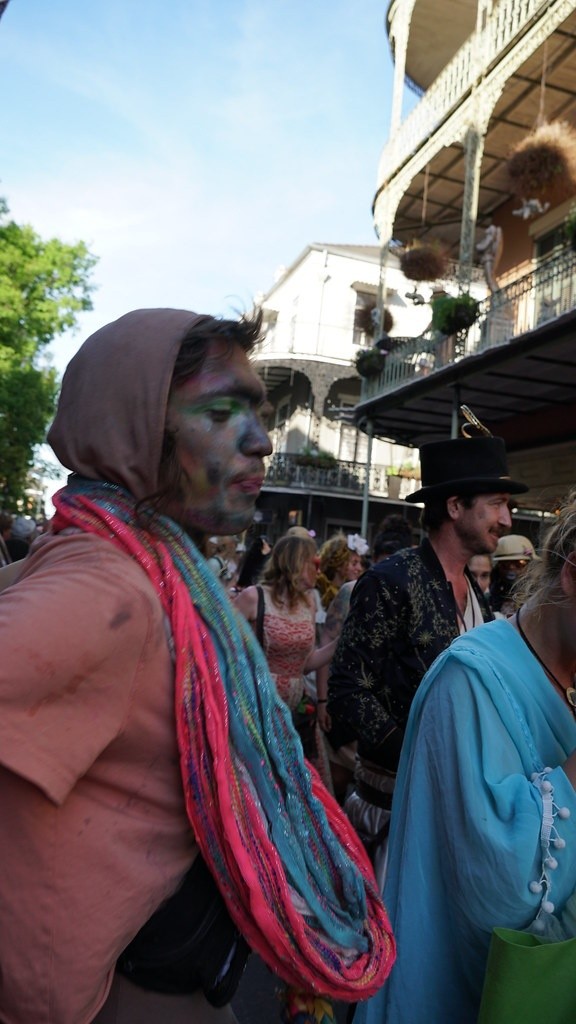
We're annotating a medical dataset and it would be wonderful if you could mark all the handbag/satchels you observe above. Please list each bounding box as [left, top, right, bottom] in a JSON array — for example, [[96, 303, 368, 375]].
[[477, 928, 576, 1024], [115, 861, 252, 1009]]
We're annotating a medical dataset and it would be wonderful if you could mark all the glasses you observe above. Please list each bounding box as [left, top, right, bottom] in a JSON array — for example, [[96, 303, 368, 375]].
[[498, 559, 529, 569]]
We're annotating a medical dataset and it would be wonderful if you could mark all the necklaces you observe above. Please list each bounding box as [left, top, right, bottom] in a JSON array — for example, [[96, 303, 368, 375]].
[[450, 575, 475, 632], [516, 610, 576, 719]]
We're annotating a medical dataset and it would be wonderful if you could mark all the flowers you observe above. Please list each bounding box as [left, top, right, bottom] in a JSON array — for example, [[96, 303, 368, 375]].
[[351, 347, 386, 365]]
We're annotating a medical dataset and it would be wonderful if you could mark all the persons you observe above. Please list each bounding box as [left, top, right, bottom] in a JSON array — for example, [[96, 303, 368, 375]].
[[467, 535, 541, 622], [206, 514, 421, 808], [352, 497, 576, 1024], [0, 513, 51, 568], [326, 406, 528, 895], [0, 308, 396, 1024]]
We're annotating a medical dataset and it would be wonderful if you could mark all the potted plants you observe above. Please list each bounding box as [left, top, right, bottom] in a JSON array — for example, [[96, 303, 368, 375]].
[[295, 447, 339, 470], [431, 292, 481, 336], [386, 463, 422, 500]]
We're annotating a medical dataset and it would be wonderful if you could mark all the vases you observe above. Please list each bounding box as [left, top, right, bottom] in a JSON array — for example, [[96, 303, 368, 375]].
[[356, 356, 385, 377]]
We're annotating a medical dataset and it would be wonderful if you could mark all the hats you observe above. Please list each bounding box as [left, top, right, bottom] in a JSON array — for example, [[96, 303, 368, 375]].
[[209, 536, 218, 544], [286, 526, 316, 539], [235, 543, 246, 551], [218, 535, 240, 544], [11, 517, 36, 537], [404, 436, 529, 503], [492, 535, 542, 561]]
